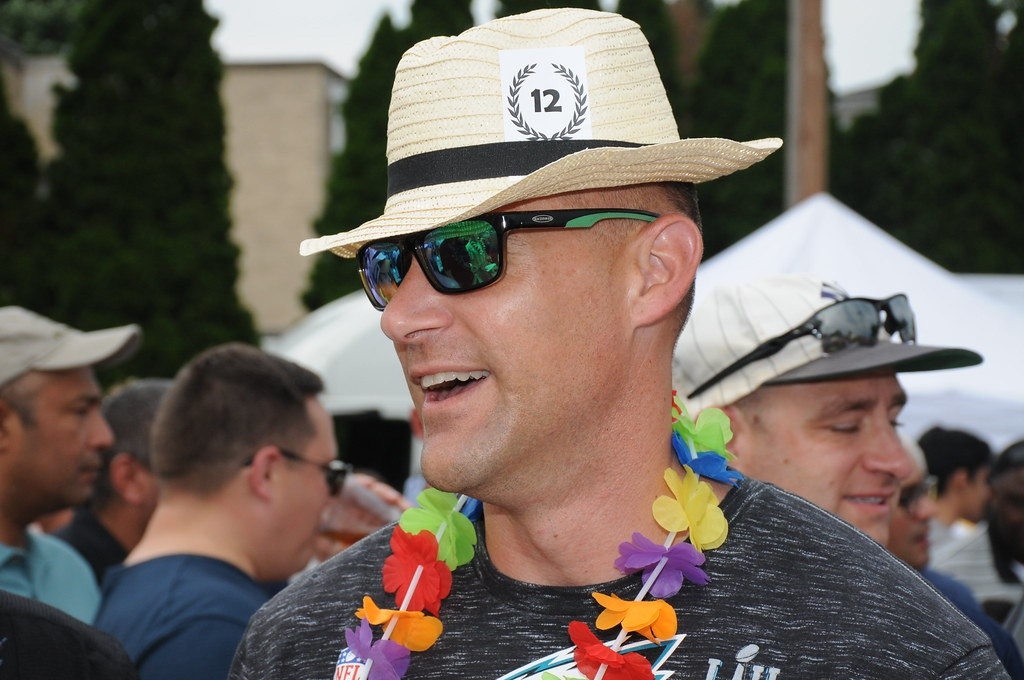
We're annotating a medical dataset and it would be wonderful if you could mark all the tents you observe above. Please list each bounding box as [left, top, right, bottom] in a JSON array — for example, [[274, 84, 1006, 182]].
[[276, 194, 1024, 475]]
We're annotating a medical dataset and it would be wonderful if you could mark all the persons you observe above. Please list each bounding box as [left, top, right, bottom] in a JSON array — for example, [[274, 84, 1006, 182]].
[[227, 9, 1006, 680], [0, 278, 1024, 680]]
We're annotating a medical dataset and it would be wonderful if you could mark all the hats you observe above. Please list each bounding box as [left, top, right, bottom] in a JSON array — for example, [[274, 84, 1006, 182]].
[[299, 8, 784, 259], [672, 275, 983, 431], [0, 305, 142, 385]]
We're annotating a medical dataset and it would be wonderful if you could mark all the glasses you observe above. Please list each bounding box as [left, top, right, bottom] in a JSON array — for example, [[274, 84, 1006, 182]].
[[899, 476, 940, 508], [242, 448, 353, 497], [686, 294, 916, 398], [355, 208, 661, 312]]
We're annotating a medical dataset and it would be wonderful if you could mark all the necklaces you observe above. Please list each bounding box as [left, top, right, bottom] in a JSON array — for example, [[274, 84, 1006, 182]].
[[345, 390, 739, 680]]
